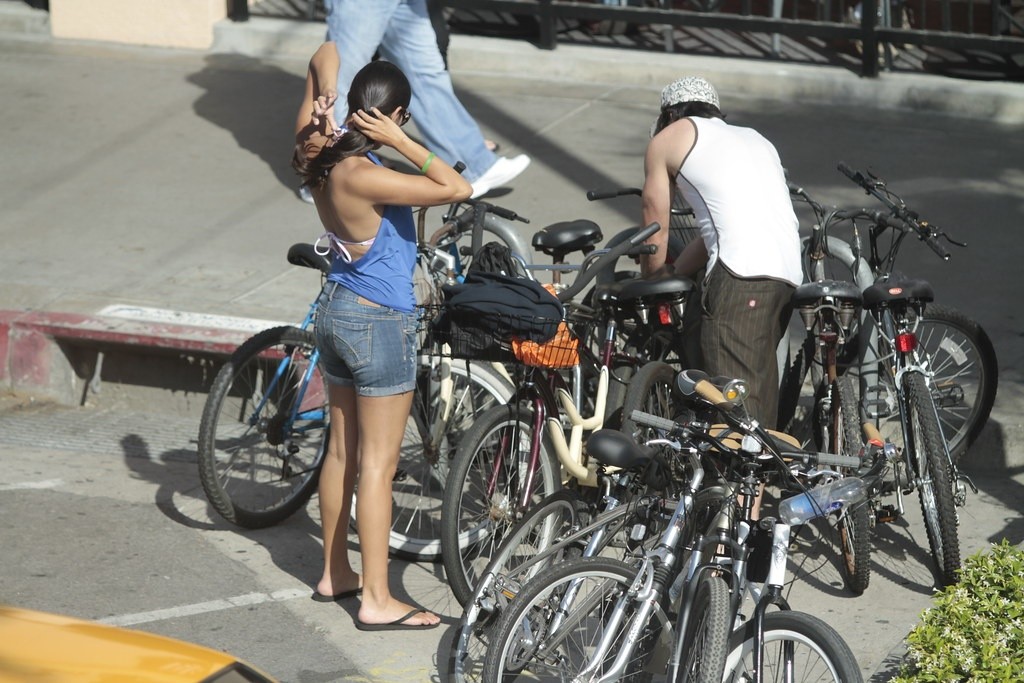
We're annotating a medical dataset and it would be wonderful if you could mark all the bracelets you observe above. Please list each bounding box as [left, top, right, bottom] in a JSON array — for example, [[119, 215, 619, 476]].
[[419, 152, 435, 174]]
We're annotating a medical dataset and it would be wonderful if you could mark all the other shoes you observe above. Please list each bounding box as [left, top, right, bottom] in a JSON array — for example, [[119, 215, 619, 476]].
[[470, 154, 530, 201], [485, 139, 498, 152], [299, 179, 315, 203]]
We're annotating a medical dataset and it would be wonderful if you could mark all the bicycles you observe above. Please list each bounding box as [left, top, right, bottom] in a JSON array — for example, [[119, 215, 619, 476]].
[[835, 162, 999, 588], [197, 160, 910, 682]]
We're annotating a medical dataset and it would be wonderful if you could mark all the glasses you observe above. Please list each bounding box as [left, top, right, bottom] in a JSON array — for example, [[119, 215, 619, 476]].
[[396, 105, 411, 127]]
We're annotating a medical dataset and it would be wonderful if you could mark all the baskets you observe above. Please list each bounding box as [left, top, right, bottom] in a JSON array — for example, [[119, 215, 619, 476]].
[[425, 305, 594, 368], [668, 188, 702, 245]]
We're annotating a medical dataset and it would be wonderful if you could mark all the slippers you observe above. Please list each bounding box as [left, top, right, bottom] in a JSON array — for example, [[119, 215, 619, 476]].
[[356, 607, 440, 631], [311, 587, 363, 602]]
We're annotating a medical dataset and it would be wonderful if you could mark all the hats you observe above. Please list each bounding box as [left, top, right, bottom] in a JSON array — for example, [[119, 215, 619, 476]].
[[650, 76, 721, 137]]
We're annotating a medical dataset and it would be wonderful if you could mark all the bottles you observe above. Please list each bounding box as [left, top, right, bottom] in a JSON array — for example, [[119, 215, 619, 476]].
[[779, 477, 870, 525]]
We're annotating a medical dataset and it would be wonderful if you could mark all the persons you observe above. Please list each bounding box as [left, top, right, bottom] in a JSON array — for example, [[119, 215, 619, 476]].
[[291, 59, 474, 626], [300, 0, 531, 206], [639, 76, 803, 542]]
